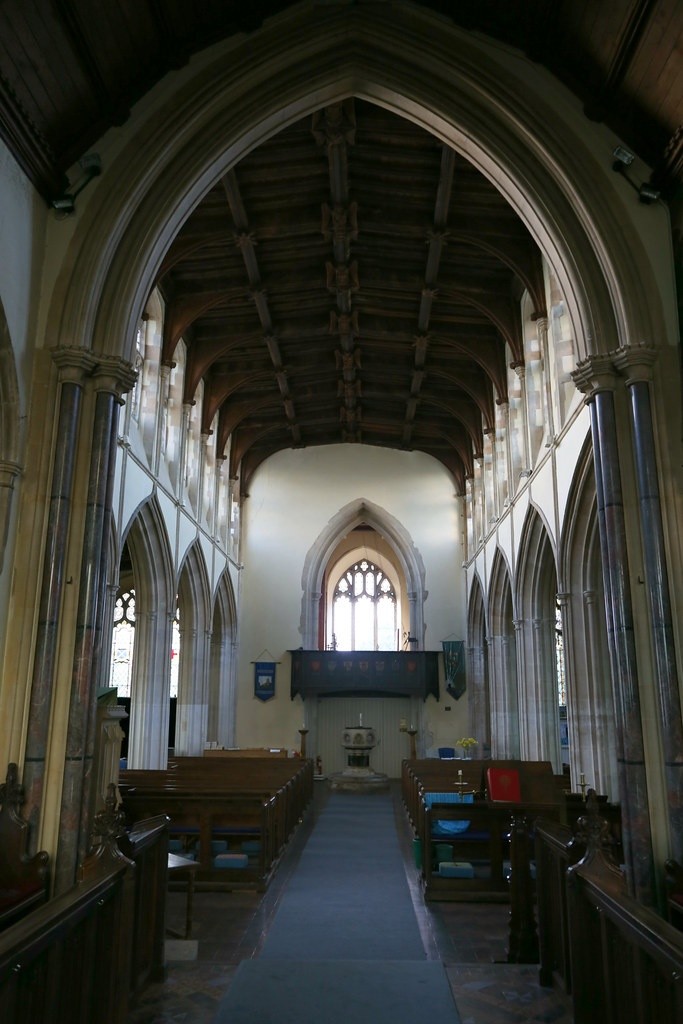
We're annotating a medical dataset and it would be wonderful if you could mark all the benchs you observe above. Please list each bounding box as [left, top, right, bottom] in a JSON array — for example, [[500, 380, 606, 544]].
[[110, 754, 314, 895], [398, 756, 610, 903]]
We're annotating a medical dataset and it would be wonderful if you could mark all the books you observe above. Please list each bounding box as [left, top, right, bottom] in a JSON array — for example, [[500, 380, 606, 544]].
[[487, 767, 522, 803]]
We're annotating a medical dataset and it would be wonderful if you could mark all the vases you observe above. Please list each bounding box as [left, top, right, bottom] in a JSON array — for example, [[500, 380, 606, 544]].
[[464, 747, 469, 757]]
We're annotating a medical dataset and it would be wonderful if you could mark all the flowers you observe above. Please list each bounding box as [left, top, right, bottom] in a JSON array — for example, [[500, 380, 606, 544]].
[[457, 736, 478, 748]]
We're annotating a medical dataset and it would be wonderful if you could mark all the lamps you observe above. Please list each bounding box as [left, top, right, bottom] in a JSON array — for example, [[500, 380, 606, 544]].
[[639, 182, 663, 206], [81, 152, 104, 177], [612, 144, 635, 175], [50, 194, 75, 217]]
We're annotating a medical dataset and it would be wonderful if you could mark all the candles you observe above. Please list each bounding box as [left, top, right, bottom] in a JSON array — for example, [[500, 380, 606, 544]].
[[411, 724, 413, 730], [303, 721, 305, 729], [458, 770, 462, 774], [581, 772, 584, 775], [360, 713, 363, 726]]
